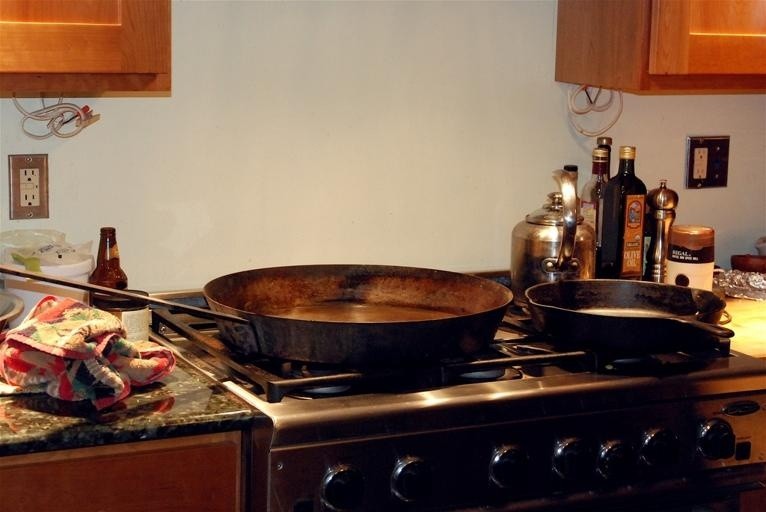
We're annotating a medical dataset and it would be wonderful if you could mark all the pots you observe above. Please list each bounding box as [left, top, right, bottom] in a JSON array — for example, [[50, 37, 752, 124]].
[[524, 279, 735, 354], [0, 263, 514, 368]]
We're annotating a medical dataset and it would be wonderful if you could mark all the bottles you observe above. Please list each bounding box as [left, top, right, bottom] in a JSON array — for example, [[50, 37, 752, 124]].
[[578, 148, 611, 279], [598, 144, 647, 281], [86, 227, 127, 321], [597, 136, 612, 178], [106, 288, 149, 342], [562, 164, 577, 198]]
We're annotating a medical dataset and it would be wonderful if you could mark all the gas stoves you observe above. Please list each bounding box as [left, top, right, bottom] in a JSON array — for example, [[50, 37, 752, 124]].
[[149, 289, 762, 509]]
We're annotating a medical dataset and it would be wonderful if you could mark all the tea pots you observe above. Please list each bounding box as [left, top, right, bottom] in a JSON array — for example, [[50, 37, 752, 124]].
[[511, 168, 597, 308], [647, 179, 679, 281]]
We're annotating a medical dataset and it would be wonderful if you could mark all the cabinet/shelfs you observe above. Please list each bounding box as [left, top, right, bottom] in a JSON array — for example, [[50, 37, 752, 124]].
[[555, 0, 766, 96], [1, 1, 172, 95]]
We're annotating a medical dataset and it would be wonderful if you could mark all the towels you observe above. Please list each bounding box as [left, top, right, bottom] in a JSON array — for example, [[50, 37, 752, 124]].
[[0, 296, 176, 410]]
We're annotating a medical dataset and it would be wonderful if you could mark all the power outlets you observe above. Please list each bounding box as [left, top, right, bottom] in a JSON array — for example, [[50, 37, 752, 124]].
[[9, 153, 49, 219]]
